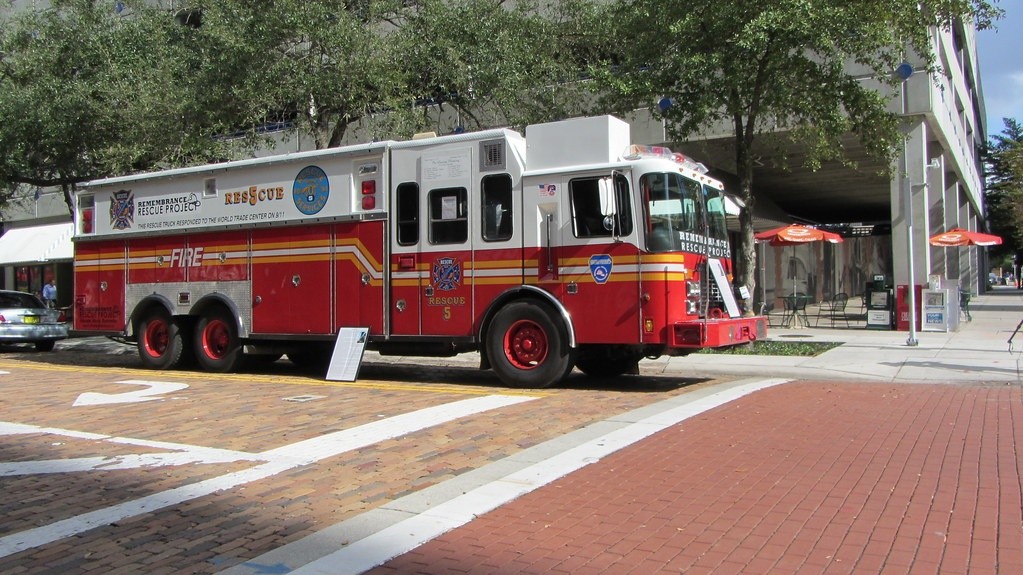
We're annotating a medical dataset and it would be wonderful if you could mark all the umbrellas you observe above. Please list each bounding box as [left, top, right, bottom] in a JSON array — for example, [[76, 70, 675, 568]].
[[753, 223, 845, 327], [930, 228, 1003, 289]]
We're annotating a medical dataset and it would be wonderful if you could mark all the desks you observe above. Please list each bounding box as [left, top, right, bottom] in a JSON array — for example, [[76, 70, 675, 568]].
[[778, 295, 813, 327]]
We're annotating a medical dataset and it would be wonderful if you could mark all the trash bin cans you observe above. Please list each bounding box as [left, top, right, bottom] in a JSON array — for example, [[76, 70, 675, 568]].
[[920, 288, 959, 332], [896, 284, 923, 332], [864, 280, 894, 332]]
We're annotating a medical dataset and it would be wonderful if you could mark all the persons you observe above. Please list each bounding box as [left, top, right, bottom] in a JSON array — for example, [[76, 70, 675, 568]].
[[1004, 272, 1010, 285], [42, 279, 58, 309]]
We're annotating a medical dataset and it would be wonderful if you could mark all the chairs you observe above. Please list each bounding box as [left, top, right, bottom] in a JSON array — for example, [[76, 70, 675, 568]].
[[754, 292, 851, 329], [859, 291, 867, 315]]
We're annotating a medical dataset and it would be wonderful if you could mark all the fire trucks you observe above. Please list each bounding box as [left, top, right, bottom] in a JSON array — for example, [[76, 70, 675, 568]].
[[71, 114, 769, 389]]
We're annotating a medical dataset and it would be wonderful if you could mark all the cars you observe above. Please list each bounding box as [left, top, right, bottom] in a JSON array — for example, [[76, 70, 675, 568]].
[[0, 289, 69, 354]]
